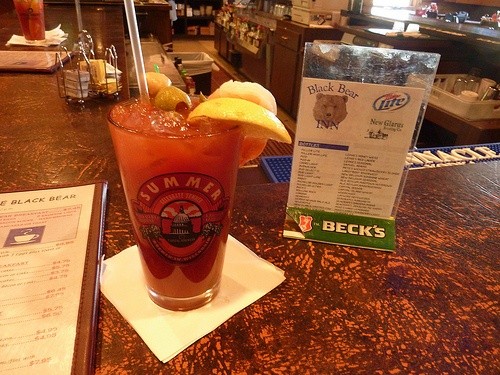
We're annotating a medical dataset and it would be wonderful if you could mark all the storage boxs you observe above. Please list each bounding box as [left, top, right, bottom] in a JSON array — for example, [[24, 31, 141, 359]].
[[291, 7, 341, 25], [406, 74, 500, 121], [291, 0, 350, 12]]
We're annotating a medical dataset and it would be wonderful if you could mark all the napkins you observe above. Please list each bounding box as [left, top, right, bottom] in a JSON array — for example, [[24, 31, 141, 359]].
[[100, 233, 286, 365], [6, 24, 69, 47]]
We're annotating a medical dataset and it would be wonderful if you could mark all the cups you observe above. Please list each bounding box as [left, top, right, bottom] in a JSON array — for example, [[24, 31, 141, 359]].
[[445, 12, 453, 23], [107, 94, 247, 312], [12, 0, 46, 43], [452, 76, 500, 101]]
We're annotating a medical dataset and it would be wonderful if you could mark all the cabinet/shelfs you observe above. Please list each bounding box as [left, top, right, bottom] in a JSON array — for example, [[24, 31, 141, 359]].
[[171, 0, 344, 121]]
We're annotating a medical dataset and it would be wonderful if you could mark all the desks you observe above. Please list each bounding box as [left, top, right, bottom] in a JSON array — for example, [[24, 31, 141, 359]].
[[371, 9, 500, 74], [337, 26, 453, 74], [420, 105, 500, 145], [134, 4, 172, 44]]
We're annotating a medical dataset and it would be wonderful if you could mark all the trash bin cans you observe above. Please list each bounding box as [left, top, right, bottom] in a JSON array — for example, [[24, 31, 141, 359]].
[[167, 51, 214, 96]]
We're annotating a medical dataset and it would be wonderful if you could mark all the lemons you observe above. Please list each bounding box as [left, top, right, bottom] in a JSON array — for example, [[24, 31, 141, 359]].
[[185, 98, 292, 144]]
